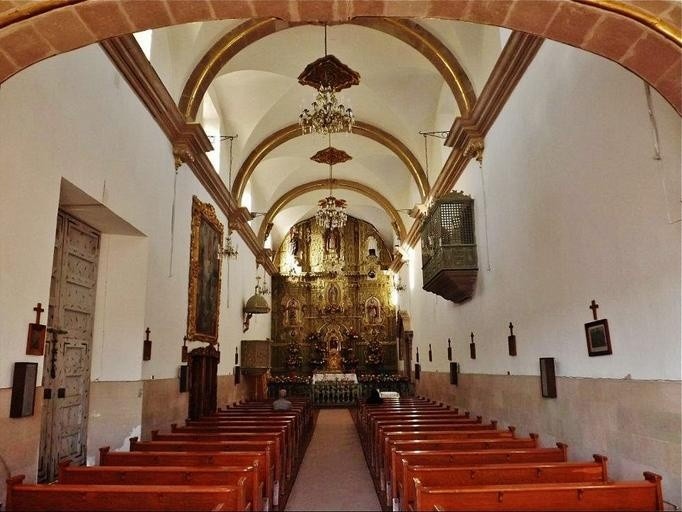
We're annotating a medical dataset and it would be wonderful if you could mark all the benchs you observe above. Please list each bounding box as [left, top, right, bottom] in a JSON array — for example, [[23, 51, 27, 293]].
[[353, 394, 668, 511], [4, 393, 319, 512]]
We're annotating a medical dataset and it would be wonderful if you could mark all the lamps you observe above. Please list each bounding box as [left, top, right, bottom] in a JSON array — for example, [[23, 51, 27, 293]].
[[295, 20, 361, 232], [243, 284, 272, 333]]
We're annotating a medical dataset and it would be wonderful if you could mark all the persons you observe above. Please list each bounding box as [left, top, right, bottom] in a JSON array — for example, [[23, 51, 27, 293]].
[[272, 389, 292, 412], [289, 298, 297, 317], [366, 389, 384, 403], [330, 328, 338, 341], [330, 283, 338, 303], [369, 299, 378, 317]]
